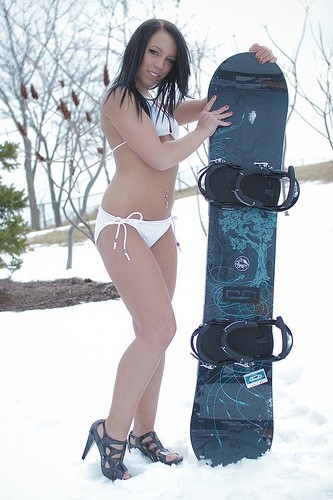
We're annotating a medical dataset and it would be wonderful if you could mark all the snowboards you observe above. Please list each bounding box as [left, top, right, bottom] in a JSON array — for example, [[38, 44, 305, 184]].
[[188, 50, 290, 469]]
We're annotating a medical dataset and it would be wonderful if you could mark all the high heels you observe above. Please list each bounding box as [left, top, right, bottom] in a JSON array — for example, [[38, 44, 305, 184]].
[[129, 431, 183, 464], [82, 419, 131, 481]]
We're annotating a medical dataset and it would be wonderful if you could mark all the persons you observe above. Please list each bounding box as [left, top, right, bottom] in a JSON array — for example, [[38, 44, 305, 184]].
[[80, 17, 278, 482]]
